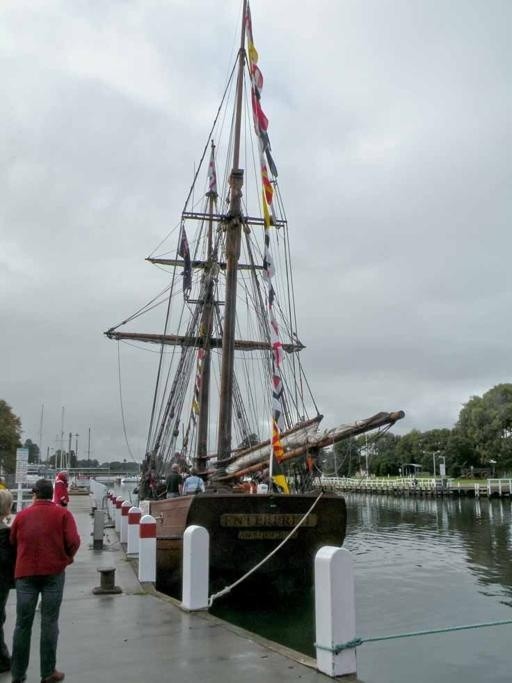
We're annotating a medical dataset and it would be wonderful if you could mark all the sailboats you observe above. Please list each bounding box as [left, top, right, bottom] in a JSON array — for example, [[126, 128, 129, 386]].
[[101, 0, 412, 591], [41, 430, 90, 495]]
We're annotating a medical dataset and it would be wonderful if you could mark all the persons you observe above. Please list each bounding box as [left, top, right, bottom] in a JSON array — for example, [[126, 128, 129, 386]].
[[9, 479, 81, 683], [182, 468, 206, 496], [175, 452, 191, 476], [0, 489, 18, 665], [149, 467, 159, 498], [166, 464, 184, 499], [0, 478, 6, 490], [54, 470, 69, 508]]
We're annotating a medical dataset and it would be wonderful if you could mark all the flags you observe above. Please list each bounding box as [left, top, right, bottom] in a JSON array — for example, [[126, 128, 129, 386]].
[[271, 475, 289, 495]]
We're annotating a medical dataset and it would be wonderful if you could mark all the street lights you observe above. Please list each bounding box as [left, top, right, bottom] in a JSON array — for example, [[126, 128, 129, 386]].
[[432, 450, 440, 476], [488, 459, 497, 478], [438, 455, 445, 464]]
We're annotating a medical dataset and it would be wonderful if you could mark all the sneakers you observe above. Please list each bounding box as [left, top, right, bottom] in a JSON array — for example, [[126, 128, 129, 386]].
[[42, 671, 64, 683]]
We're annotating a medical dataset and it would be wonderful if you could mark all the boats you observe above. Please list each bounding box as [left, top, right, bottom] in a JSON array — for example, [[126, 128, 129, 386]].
[[121, 474, 142, 482]]
